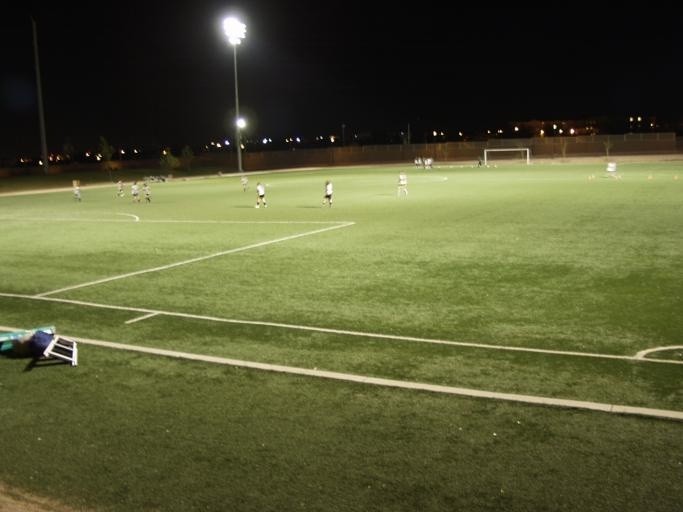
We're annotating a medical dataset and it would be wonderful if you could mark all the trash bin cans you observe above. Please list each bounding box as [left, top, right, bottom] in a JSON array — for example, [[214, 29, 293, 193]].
[[71, 179, 80, 187]]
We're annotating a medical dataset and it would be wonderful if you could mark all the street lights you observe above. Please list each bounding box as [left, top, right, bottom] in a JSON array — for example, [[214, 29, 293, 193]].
[[222, 16, 248, 173]]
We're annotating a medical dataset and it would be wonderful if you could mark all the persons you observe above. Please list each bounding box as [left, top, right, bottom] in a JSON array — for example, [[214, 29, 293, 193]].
[[255, 183, 267, 209], [606, 158, 617, 181], [397, 172, 408, 198], [415, 157, 433, 170], [322, 180, 333, 207], [71, 170, 222, 204], [477, 156, 482, 168], [240, 175, 249, 193]]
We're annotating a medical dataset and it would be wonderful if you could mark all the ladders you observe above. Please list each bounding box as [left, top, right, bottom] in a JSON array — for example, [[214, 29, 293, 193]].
[[43, 335, 78, 366]]
